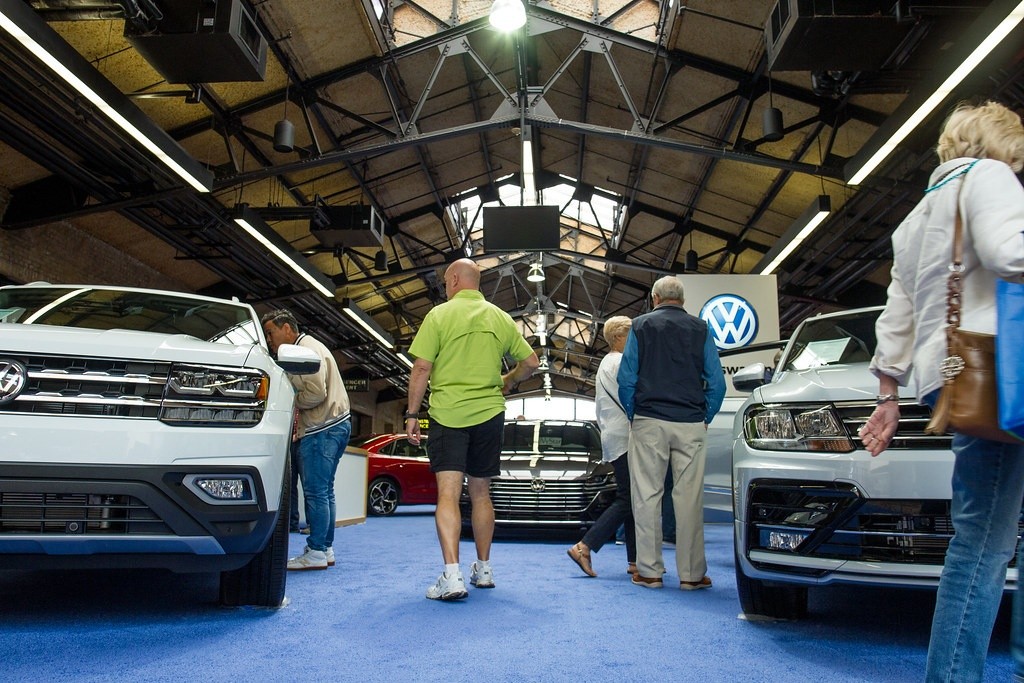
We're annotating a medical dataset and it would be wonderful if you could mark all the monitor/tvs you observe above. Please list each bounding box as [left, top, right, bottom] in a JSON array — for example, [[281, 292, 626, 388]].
[[482, 206, 560, 253]]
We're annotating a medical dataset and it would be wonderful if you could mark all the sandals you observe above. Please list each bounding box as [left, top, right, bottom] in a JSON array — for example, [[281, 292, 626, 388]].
[[567, 544, 597, 577], [627, 562, 667, 573]]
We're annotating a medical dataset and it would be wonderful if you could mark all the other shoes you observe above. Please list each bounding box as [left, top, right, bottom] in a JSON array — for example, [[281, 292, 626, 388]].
[[301, 527, 311, 534], [291, 529, 300, 533]]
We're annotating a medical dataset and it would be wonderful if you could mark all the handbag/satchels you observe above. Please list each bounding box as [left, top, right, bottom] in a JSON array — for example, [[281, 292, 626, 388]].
[[994, 279, 1024, 441], [924, 158, 1024, 445]]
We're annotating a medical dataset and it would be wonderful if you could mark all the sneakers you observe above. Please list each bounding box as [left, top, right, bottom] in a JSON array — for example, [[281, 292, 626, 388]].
[[631, 574, 663, 588], [469, 562, 496, 587], [680, 576, 713, 590], [324, 546, 335, 566], [287, 546, 327, 570], [426, 571, 469, 600]]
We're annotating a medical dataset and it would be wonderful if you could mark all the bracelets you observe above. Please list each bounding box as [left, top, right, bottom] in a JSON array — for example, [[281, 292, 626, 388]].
[[877, 394, 899, 403]]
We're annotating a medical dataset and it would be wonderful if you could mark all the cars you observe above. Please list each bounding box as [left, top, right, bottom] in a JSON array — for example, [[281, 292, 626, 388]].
[[346, 432, 438, 517], [459, 419, 618, 544]]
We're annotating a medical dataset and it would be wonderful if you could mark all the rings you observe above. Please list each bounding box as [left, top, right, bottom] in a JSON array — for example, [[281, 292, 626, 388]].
[[409, 439, 410, 440]]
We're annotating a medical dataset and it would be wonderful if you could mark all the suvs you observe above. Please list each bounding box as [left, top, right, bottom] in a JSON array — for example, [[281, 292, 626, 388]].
[[0, 280, 321, 608], [702, 305, 1019, 623]]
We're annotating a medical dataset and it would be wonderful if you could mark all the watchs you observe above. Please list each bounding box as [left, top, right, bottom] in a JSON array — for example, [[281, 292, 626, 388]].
[[406, 410, 419, 418]]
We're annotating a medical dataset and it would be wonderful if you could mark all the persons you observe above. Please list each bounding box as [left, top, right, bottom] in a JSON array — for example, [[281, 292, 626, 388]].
[[568, 316, 665, 575], [261, 310, 351, 569], [858, 103, 1024, 683], [616, 276, 727, 591], [407, 259, 539, 600]]
